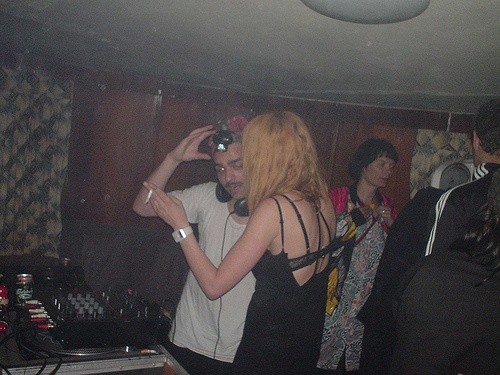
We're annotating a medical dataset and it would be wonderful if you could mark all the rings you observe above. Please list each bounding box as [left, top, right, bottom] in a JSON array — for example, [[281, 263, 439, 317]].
[[383, 210, 385, 213]]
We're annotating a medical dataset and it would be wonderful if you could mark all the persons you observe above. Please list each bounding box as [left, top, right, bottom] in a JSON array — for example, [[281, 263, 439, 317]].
[[141, 107, 338, 375], [422, 99, 500, 257], [131, 124, 257, 374], [355, 185, 454, 374], [313, 138, 399, 375], [395, 203, 500, 375]]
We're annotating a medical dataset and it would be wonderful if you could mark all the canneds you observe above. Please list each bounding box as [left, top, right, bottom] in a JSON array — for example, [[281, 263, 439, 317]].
[[14, 273, 34, 307]]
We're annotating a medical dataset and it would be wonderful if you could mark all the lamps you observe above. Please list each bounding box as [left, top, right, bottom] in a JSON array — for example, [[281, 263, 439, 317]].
[[302, 0, 430, 25]]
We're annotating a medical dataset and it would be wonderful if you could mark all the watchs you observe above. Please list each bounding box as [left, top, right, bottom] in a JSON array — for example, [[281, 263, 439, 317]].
[[171, 226, 193, 243]]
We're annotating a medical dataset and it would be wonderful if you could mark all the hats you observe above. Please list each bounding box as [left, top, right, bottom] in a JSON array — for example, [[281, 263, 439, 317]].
[[208, 115, 250, 157]]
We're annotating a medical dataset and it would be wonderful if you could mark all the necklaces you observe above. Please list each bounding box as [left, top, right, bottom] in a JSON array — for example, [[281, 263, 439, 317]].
[[368, 198, 376, 210]]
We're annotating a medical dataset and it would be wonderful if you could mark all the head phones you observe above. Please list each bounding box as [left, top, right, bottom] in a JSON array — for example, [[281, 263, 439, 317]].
[[215, 182, 249, 217]]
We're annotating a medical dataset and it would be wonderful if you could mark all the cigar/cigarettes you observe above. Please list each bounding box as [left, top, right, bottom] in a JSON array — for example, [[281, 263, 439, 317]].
[[144, 188, 153, 204]]
